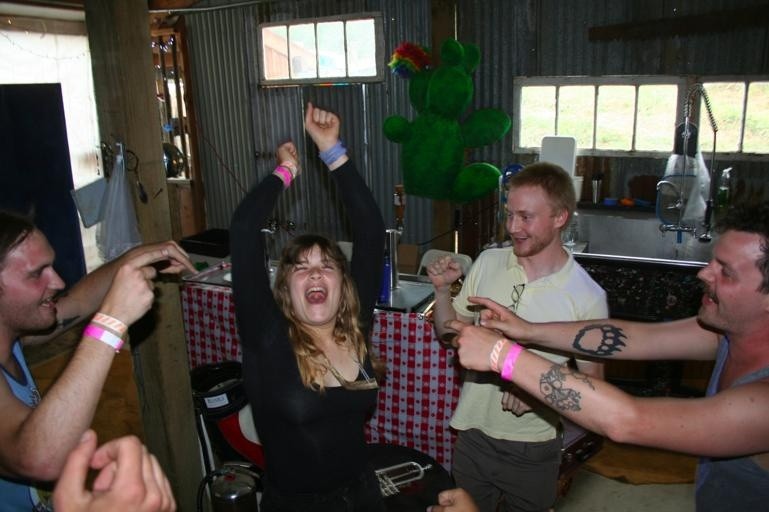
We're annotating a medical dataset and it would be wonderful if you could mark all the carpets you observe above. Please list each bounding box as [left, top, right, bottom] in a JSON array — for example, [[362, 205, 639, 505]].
[[567, 440, 699, 487]]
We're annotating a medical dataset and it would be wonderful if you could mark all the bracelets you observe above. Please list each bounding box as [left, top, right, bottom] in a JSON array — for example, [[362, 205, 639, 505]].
[[500, 342, 524, 382], [279, 161, 298, 180], [81, 324, 125, 353], [489, 337, 509, 375], [319, 142, 348, 166], [92, 311, 127, 336], [272, 166, 291, 192]]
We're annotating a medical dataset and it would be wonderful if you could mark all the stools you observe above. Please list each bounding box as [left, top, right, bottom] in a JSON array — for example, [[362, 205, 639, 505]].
[[178, 225, 230, 272]]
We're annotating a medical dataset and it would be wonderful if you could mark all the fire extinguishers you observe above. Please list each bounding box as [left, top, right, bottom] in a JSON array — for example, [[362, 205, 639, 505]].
[[197, 463, 264, 512]]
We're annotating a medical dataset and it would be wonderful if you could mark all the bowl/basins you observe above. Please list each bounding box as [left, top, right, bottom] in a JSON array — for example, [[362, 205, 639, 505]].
[[189, 359, 248, 420], [603, 199, 617, 206]]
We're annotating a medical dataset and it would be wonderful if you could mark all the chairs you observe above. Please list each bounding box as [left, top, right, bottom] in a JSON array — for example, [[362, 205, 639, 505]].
[[186, 352, 268, 510]]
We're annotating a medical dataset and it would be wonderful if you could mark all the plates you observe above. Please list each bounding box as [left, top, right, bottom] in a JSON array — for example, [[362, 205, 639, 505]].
[[162, 142, 184, 180]]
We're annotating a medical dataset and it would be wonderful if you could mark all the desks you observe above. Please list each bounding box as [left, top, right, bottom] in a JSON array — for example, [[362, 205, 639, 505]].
[[506, 207, 725, 398], [257, 443, 457, 511], [171, 256, 465, 479]]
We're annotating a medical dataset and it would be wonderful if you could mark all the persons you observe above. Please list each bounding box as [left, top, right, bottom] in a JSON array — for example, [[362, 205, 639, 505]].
[[50, 429, 179, 512], [229, 100, 387, 512], [427, 486, 479, 511], [426, 161, 611, 512], [443, 199, 768, 511], [2, 210, 199, 512]]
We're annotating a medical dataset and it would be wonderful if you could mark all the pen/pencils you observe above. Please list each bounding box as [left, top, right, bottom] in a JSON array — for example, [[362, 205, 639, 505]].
[[191, 263, 229, 280]]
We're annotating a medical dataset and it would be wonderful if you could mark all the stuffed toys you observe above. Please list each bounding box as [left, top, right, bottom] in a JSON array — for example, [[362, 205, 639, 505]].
[[382, 38, 513, 207]]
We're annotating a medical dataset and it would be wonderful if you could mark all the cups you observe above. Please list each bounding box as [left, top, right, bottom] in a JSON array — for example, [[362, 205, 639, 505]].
[[591, 174, 604, 206]]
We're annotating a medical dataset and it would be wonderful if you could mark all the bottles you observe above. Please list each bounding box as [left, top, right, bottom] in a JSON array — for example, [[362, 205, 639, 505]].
[[379, 250, 391, 305]]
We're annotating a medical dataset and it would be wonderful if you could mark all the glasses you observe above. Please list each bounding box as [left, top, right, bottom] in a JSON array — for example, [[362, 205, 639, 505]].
[[506, 283, 525, 315]]
